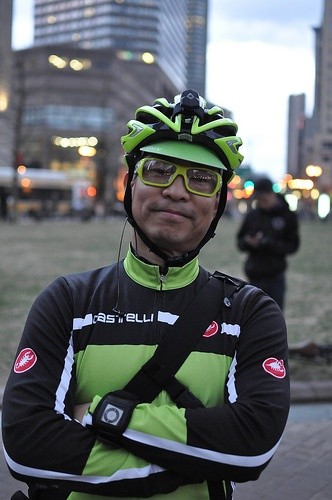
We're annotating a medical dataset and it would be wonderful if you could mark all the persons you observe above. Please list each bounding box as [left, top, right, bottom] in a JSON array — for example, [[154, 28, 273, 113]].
[[237, 178, 301, 310], [1, 89, 291, 500]]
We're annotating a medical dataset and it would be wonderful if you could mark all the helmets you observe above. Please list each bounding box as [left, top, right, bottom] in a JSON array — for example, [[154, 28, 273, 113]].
[[121, 89, 244, 187]]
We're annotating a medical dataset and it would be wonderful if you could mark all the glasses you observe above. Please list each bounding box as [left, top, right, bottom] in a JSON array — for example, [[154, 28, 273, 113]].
[[132, 157, 223, 197]]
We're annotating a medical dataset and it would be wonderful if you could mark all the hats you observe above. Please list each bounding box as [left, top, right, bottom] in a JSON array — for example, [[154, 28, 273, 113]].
[[251, 179, 273, 199]]
